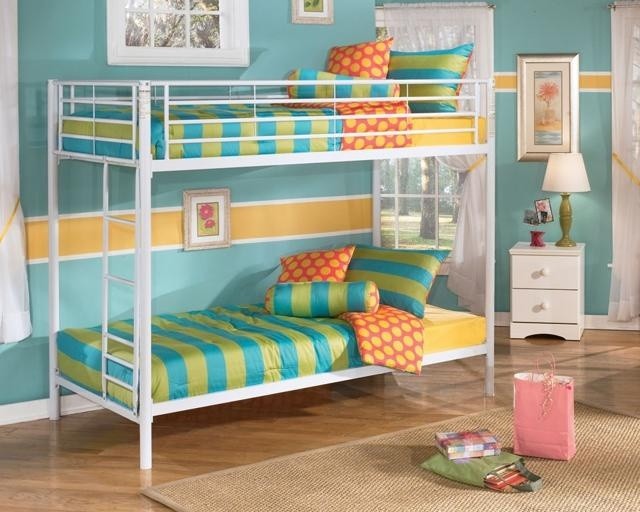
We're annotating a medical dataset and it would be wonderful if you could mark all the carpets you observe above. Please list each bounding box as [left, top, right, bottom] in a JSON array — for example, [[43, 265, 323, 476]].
[[137, 397, 639, 512]]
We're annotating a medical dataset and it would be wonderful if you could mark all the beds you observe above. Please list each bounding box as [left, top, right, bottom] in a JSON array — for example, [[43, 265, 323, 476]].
[[47, 78, 497, 470]]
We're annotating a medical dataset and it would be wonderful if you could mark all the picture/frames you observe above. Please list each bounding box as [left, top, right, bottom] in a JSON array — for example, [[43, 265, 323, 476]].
[[181, 188, 231, 251], [291, 0, 333, 24], [534, 197, 554, 224], [517, 52, 582, 164]]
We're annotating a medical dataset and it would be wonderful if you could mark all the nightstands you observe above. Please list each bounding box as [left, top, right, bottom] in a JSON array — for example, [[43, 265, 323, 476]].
[[507, 241, 588, 341]]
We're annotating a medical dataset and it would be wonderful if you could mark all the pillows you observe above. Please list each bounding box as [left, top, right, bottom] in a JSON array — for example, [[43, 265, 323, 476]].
[[387, 42, 475, 113], [265, 280, 380, 317], [278, 244, 356, 283], [344, 244, 452, 319], [286, 68, 401, 104], [325, 37, 394, 80]]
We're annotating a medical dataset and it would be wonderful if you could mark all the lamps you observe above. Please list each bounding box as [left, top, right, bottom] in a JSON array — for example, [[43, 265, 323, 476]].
[[540, 152, 592, 247]]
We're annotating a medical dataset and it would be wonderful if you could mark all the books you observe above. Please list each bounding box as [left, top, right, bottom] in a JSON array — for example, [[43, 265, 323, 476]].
[[485, 462, 533, 492]]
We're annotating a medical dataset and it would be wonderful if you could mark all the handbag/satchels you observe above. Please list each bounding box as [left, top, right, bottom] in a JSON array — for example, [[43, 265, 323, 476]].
[[512, 350, 577, 461]]
